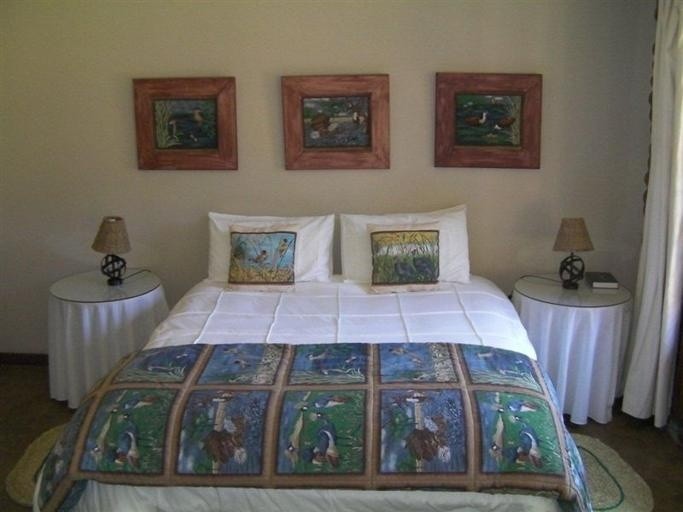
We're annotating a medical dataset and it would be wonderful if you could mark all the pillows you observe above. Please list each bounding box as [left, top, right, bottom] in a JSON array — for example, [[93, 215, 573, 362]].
[[208, 204, 470, 295]]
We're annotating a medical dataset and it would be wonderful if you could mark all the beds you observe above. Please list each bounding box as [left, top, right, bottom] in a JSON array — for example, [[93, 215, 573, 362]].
[[32, 275, 593, 512]]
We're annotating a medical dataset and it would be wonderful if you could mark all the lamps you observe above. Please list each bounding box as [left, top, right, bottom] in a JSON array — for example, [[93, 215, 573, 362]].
[[91, 216, 132, 286], [553, 218, 594, 289]]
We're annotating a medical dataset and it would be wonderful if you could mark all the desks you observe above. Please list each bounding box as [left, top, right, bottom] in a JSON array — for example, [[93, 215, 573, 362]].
[[512, 273, 634, 426], [47, 268, 168, 409]]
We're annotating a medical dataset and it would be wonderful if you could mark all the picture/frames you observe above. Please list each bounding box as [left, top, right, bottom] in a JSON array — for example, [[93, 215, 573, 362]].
[[435, 72, 542, 169], [281, 75, 391, 170], [133, 77, 238, 170]]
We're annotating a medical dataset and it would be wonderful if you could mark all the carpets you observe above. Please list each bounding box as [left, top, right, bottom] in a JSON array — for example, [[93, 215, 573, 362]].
[[5, 424, 65, 507], [570, 433, 655, 512]]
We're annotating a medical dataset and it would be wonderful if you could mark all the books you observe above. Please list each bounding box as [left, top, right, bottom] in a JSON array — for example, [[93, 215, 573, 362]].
[[587, 271, 618, 289]]
